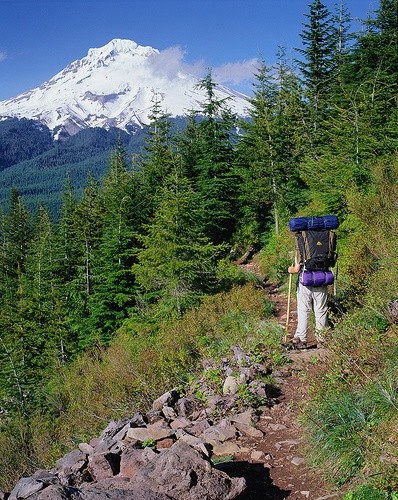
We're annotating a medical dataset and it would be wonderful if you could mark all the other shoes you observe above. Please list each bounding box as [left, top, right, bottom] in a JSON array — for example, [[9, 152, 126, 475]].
[[316, 340, 326, 349], [283, 338, 307, 349]]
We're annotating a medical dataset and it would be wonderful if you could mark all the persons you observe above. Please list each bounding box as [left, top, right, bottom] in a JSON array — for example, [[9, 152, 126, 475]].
[[287, 215, 341, 349]]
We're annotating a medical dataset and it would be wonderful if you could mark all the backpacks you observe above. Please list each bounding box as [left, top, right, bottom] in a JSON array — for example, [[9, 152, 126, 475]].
[[294, 229, 336, 270]]
